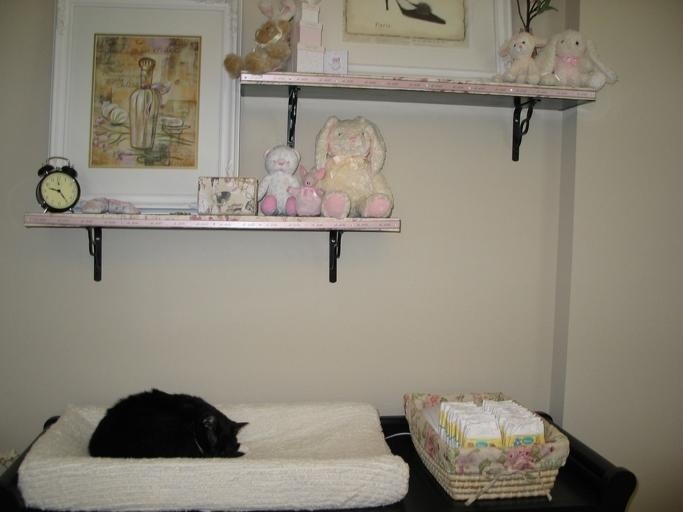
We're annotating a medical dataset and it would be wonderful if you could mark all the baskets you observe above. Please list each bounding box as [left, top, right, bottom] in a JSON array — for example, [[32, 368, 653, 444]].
[[404, 392, 570, 506]]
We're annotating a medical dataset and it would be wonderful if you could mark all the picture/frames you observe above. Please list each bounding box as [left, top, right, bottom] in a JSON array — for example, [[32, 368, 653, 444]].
[[318, 1, 514, 87], [46, 0, 243, 210]]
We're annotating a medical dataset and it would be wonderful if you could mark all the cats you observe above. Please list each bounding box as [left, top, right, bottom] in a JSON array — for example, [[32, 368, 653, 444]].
[[88, 389, 248, 458]]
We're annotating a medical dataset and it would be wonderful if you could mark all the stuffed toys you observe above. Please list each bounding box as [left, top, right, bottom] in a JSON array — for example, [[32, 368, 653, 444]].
[[286, 165, 326, 215], [314, 116, 393, 219], [492, 33, 548, 82], [222, 1, 295, 79], [535, 29, 616, 91], [256, 146, 300, 217]]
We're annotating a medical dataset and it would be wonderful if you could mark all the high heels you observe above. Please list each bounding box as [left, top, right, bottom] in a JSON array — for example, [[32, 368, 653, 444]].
[[386, 0, 446, 26]]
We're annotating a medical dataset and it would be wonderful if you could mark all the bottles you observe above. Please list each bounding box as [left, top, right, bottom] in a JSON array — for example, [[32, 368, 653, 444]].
[[130, 57, 161, 149]]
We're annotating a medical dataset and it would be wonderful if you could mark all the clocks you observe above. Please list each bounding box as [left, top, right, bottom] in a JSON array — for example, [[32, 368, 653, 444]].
[[34, 156, 80, 214]]
